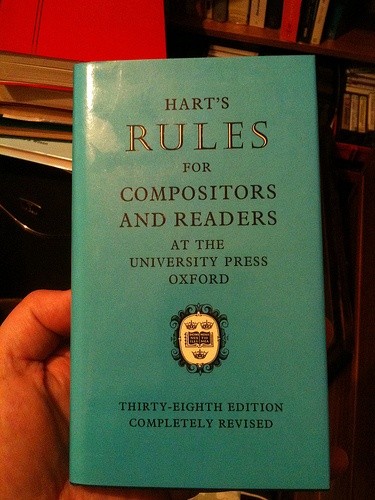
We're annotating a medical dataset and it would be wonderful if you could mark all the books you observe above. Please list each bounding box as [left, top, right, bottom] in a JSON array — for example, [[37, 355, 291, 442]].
[[0, 1, 375, 173], [72, 55, 333, 486]]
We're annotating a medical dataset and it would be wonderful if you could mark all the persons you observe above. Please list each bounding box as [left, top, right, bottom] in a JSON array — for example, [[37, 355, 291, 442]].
[[0, 289, 349, 500]]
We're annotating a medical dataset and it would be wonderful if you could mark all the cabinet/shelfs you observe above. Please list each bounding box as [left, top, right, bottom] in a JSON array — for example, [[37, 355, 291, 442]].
[[167, 8, 375, 500]]
